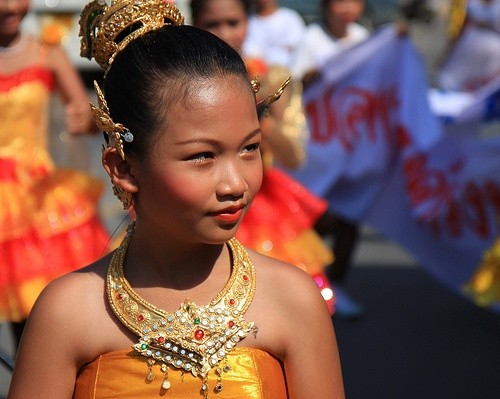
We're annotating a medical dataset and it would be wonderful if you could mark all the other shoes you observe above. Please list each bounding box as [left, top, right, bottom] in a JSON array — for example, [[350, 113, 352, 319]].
[[329, 283, 360, 318]]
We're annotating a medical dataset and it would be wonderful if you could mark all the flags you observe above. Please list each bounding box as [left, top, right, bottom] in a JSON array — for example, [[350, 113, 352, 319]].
[[271, 22, 500, 310]]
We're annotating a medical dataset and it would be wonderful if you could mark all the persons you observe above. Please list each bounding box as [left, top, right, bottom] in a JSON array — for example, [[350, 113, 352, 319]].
[[245, 0, 370, 316], [6, 0, 346, 399], [0, 0, 112, 324], [110, 0, 334, 314]]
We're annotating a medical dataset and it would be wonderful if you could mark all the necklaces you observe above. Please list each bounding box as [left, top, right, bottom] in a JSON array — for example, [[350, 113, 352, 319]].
[[104, 216, 257, 399]]
